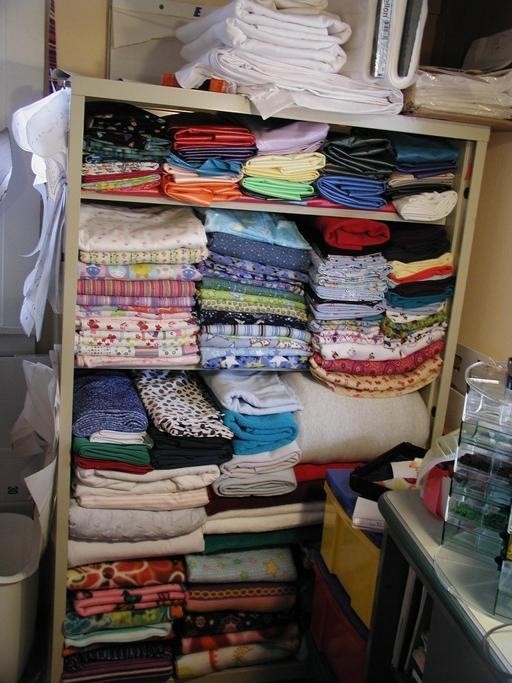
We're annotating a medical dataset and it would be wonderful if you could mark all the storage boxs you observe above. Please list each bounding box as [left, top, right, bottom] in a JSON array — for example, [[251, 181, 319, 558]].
[[321, 468, 387, 631], [311, 553, 366, 682]]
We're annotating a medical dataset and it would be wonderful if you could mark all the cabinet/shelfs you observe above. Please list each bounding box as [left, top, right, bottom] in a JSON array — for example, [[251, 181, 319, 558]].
[[51, 77, 489, 683], [365, 490, 512, 682]]
